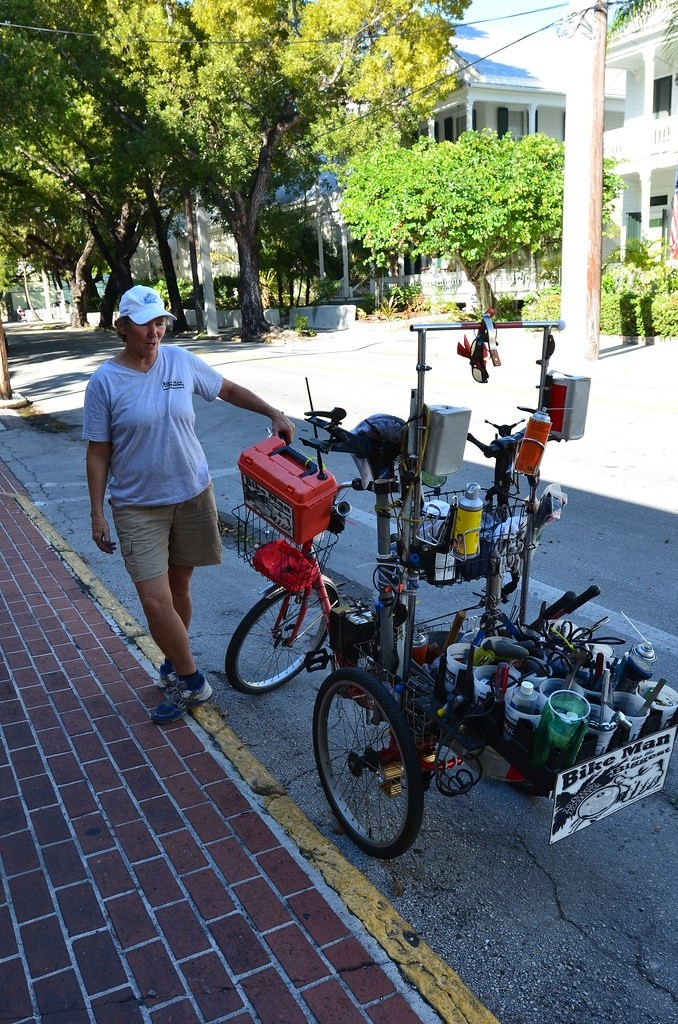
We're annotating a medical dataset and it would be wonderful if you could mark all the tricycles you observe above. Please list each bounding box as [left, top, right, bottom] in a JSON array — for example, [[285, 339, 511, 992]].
[[222, 406, 678, 861]]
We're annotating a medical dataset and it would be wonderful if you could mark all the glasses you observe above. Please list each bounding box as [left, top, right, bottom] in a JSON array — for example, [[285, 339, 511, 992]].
[[470, 339, 489, 384]]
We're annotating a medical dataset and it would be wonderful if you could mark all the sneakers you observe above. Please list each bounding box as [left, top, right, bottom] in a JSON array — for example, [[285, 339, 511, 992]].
[[151, 674, 212, 723], [157, 665, 179, 700]]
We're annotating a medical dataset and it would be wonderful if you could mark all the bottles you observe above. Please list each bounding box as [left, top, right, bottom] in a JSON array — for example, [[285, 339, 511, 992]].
[[514, 407, 553, 476], [614, 642, 657, 695], [511, 680, 538, 714], [411, 632, 429, 666], [454, 482, 484, 555]]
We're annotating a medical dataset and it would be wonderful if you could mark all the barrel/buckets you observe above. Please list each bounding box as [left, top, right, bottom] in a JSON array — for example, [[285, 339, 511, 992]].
[[444, 620, 678, 755]]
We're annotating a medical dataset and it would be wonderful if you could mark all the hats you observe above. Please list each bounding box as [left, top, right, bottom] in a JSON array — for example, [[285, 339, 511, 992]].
[[119, 285, 177, 325]]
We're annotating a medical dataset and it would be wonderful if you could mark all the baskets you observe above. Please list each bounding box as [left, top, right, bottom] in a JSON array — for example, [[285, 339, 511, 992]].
[[351, 604, 499, 752], [420, 486, 533, 587], [231, 503, 339, 595]]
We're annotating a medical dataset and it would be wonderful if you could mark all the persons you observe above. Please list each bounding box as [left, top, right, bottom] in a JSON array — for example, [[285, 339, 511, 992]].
[[17, 306, 22, 323], [81, 284, 295, 724]]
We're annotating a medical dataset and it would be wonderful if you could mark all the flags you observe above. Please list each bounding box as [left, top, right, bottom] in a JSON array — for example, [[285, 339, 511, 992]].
[[671, 166, 678, 259]]
